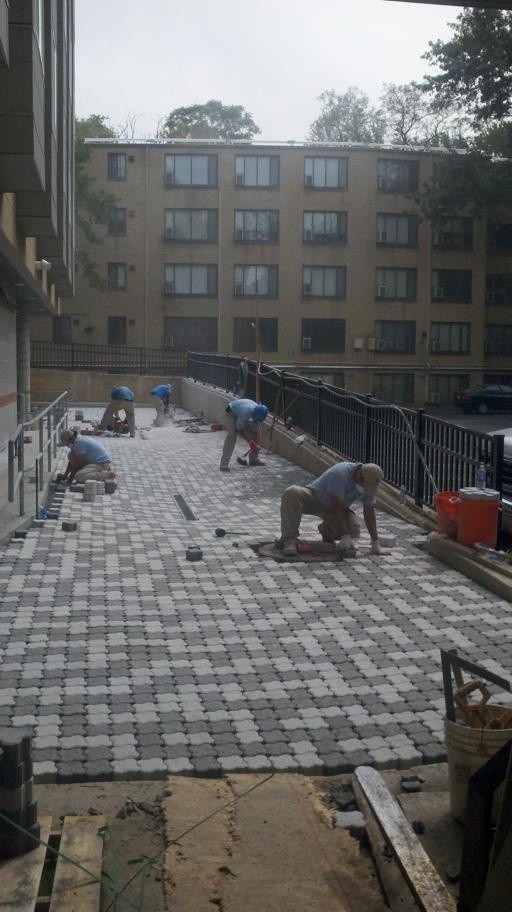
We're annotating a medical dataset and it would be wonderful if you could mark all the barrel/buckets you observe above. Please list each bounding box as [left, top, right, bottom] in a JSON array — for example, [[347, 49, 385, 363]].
[[444, 705, 512, 827], [436, 491, 462, 535], [459, 487, 500, 550]]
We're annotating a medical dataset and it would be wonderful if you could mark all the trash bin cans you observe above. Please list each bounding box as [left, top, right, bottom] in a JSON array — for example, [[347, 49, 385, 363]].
[[434, 487, 504, 554]]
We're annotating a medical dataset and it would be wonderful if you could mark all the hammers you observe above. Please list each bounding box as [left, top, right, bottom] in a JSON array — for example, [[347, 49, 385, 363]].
[[215, 528, 250, 538]]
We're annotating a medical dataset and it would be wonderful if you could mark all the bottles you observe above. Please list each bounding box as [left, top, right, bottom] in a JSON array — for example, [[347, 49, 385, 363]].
[[399, 483, 407, 506], [476, 462, 487, 492], [39, 506, 48, 520]]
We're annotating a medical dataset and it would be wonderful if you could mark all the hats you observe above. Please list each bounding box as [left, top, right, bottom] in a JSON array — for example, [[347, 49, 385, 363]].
[[359, 462, 384, 495]]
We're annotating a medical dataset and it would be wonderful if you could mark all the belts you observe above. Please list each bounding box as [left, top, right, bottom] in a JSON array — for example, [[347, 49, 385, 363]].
[[124, 399, 135, 402]]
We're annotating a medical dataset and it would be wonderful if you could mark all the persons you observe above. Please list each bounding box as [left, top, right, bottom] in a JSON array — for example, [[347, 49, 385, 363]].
[[272, 461, 392, 557], [149, 382, 175, 427], [93, 383, 136, 438], [218, 398, 270, 471], [55, 428, 116, 486]]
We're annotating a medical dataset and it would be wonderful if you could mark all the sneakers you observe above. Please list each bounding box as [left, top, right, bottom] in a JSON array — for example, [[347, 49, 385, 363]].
[[248, 456, 267, 467], [283, 538, 298, 557], [317, 523, 338, 549], [219, 462, 233, 472]]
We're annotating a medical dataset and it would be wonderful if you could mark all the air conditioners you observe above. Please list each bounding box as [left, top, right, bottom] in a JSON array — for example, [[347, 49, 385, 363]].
[[302, 337, 311, 349], [432, 340, 442, 352], [379, 176, 388, 190], [377, 285, 388, 297], [165, 228, 171, 239], [305, 174, 313, 185], [164, 172, 172, 184], [432, 232, 446, 245], [433, 286, 443, 299], [302, 283, 312, 295], [166, 335, 174, 347], [235, 174, 244, 184], [378, 231, 389, 243], [429, 391, 440, 403], [303, 229, 313, 241], [376, 339, 386, 351], [487, 292, 496, 304], [165, 280, 174, 293], [234, 286, 241, 296]]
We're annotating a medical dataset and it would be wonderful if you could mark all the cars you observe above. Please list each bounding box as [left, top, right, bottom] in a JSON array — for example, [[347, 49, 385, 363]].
[[450, 384, 512, 414], [481, 427, 512, 482]]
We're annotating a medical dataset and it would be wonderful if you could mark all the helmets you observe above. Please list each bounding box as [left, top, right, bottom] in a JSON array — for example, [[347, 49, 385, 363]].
[[253, 405, 269, 421], [60, 429, 74, 444]]
